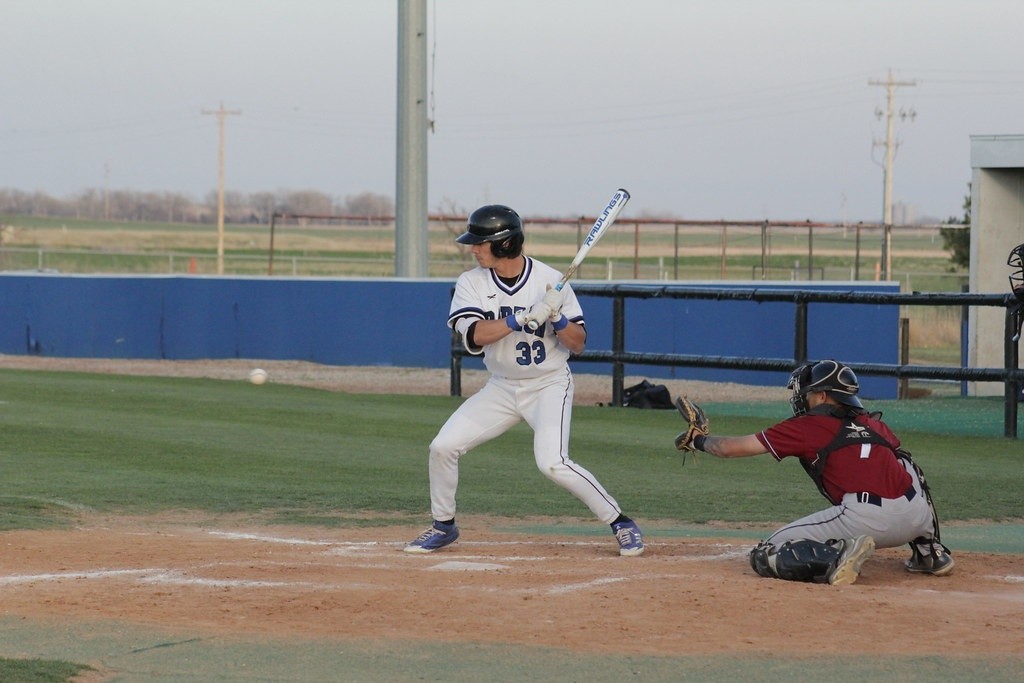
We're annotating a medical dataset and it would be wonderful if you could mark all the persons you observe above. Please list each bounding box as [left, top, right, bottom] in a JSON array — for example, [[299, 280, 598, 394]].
[[673, 358, 954, 584], [404, 204, 646, 558]]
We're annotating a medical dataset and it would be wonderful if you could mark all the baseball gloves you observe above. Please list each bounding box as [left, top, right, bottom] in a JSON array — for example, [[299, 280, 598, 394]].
[[674, 391, 710, 467]]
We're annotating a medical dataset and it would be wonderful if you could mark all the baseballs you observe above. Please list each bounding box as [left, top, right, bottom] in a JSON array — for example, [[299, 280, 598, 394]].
[[249, 368, 267, 385]]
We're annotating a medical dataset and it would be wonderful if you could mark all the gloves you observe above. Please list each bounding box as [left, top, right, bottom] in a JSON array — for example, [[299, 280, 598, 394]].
[[541, 283, 564, 323], [514, 302, 551, 328]]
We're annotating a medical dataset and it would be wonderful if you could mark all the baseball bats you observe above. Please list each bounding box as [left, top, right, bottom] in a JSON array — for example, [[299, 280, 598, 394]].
[[527, 188, 631, 331]]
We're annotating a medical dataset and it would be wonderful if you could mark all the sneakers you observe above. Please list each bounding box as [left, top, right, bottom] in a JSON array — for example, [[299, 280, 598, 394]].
[[404, 521, 460, 554], [610, 514, 645, 558], [827, 533, 876, 586], [904, 548, 955, 576]]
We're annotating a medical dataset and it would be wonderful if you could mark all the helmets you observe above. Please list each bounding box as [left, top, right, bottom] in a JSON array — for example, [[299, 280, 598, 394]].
[[786, 359, 864, 410], [453, 205, 527, 261]]
